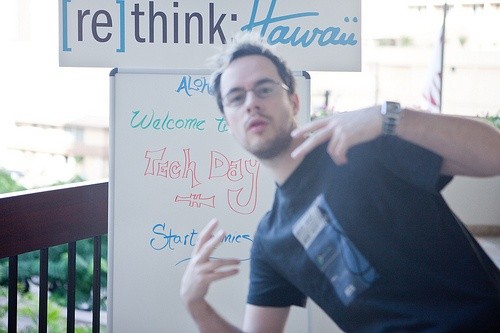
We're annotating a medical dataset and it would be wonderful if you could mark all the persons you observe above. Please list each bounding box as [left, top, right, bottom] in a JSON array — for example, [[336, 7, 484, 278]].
[[181, 41, 500, 333]]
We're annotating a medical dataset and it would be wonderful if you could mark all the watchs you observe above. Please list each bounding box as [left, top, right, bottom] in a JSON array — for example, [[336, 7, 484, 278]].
[[381, 101, 401, 136]]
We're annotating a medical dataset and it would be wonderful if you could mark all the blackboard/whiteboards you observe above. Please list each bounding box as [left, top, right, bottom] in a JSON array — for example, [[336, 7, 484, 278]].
[[103, 67, 312, 332], [440, 175, 500, 238]]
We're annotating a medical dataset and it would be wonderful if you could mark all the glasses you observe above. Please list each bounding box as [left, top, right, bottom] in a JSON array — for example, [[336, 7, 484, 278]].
[[223, 79, 290, 107]]
